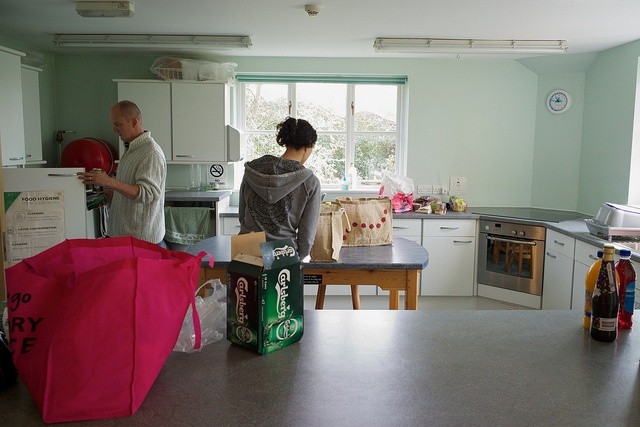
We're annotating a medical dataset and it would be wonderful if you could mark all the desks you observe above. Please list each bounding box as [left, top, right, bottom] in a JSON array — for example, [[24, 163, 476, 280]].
[[183, 235, 430, 310], [0, 309, 640, 425]]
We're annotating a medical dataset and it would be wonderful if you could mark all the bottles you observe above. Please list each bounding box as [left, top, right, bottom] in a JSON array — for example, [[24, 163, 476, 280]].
[[342, 176, 348, 190], [347, 161, 358, 189], [589, 242, 621, 343], [582, 249, 620, 331], [614, 249, 637, 330]]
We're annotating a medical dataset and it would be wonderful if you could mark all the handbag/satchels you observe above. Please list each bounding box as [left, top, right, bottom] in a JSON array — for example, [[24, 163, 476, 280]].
[[379, 177, 413, 213], [311, 201, 351, 263], [336, 197, 393, 247], [5, 236, 214, 424]]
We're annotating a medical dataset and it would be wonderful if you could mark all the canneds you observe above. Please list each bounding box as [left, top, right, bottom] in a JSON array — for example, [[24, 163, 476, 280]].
[[87, 193, 107, 211], [92, 168, 103, 193]]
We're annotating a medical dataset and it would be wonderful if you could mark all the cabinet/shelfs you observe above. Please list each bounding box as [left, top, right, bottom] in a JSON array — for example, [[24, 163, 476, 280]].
[[421, 218, 479, 296], [541, 228, 576, 309], [1, 164, 88, 267], [113, 77, 234, 165], [20, 62, 47, 163], [0, 46, 28, 168], [377, 218, 422, 296], [220, 217, 242, 235], [572, 240, 640, 307]]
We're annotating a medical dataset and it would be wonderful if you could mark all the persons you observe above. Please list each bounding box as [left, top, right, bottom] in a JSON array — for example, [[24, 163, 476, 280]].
[[235, 115, 321, 264], [77, 100, 168, 249]]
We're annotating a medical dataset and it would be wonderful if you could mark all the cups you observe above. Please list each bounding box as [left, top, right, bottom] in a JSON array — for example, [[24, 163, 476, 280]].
[[186, 163, 203, 191]]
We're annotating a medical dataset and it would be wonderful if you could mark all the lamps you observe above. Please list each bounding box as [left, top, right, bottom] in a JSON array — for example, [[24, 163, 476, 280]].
[[52, 33, 253, 49], [373, 37, 569, 56], [75, 2, 135, 18], [304, 4, 321, 17]]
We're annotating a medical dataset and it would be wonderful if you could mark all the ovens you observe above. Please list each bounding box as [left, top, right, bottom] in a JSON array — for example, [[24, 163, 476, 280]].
[[477, 218, 546, 297], [162, 200, 217, 252]]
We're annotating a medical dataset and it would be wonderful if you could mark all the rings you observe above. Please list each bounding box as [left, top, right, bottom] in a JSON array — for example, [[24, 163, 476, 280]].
[[88, 176, 93, 181]]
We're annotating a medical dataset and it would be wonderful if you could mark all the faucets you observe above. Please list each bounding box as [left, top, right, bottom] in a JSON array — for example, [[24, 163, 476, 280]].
[[320, 192, 327, 201]]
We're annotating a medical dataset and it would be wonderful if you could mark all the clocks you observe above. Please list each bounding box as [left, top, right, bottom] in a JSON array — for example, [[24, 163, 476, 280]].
[[546, 89, 571, 114]]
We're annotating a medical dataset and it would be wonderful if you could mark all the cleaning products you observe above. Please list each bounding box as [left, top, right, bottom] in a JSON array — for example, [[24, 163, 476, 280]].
[[348, 163, 357, 188], [341, 175, 349, 190]]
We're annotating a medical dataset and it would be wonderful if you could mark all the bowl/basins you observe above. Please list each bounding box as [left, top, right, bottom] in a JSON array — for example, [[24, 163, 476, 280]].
[[592, 200, 639, 226], [450, 204, 467, 211]]
